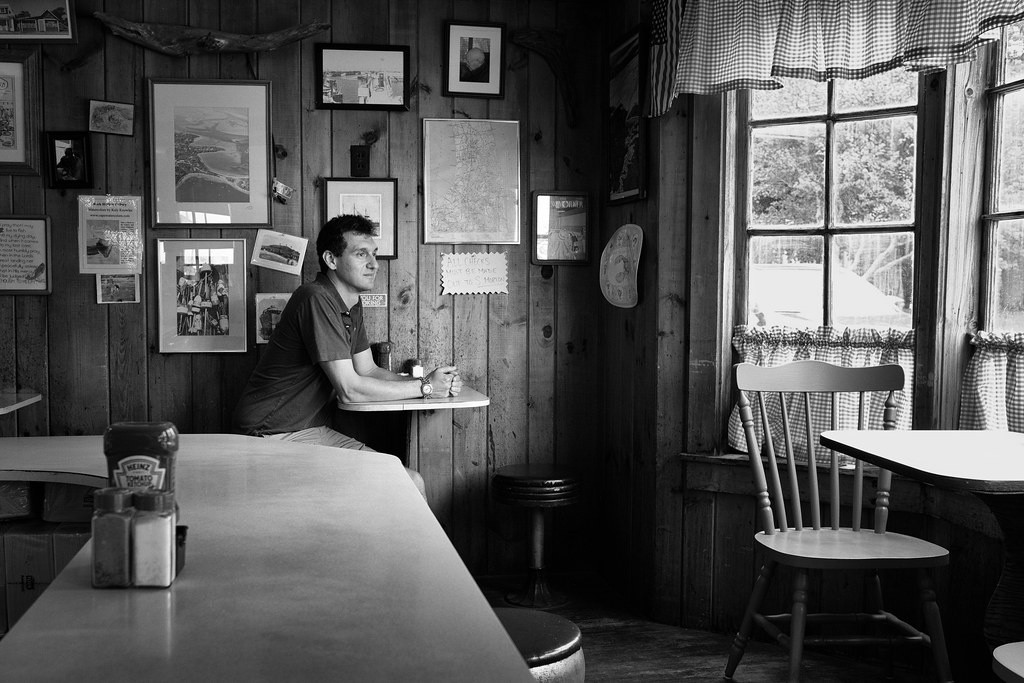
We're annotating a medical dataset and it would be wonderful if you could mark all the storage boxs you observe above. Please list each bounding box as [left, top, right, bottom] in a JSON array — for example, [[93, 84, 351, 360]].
[[0, 481, 95, 640]]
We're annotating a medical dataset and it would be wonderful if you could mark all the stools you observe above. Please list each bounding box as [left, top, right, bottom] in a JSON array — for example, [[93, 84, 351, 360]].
[[493, 465, 580, 610], [493, 606, 586, 683]]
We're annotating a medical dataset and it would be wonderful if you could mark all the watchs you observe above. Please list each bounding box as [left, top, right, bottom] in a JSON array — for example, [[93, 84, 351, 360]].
[[418, 377, 433, 400]]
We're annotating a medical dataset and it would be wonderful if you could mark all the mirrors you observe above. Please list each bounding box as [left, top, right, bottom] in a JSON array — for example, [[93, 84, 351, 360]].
[[41, 130, 95, 189]]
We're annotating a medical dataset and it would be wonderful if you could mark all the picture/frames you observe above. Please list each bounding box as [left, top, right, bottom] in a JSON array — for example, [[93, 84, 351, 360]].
[[422, 118, 521, 245], [250, 228, 309, 275], [531, 190, 590, 267], [89, 99, 135, 137], [314, 42, 409, 112], [602, 23, 647, 207], [322, 176, 398, 260], [96, 274, 140, 303], [156, 238, 248, 352], [0, 0, 78, 45], [148, 77, 274, 229], [0, 44, 44, 177], [442, 19, 506, 100]]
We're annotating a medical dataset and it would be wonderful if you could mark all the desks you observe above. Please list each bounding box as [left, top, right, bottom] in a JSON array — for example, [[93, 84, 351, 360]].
[[336, 385, 490, 412], [0, 393, 42, 414], [819, 430, 1024, 652]]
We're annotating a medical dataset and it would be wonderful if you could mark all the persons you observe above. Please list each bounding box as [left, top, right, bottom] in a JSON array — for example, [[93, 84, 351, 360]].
[[234, 215, 463, 504], [460, 48, 490, 84]]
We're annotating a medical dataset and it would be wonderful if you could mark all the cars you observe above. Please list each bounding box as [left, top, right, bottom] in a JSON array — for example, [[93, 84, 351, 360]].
[[744, 262, 913, 333]]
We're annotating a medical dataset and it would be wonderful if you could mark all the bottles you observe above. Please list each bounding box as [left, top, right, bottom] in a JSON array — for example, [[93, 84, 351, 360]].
[[130, 489, 177, 588], [103, 421, 179, 511], [90, 485, 136, 589], [378, 342, 391, 370], [411, 359, 423, 378]]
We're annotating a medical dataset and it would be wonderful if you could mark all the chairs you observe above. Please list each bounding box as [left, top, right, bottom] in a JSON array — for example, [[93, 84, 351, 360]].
[[724, 360, 953, 683]]
